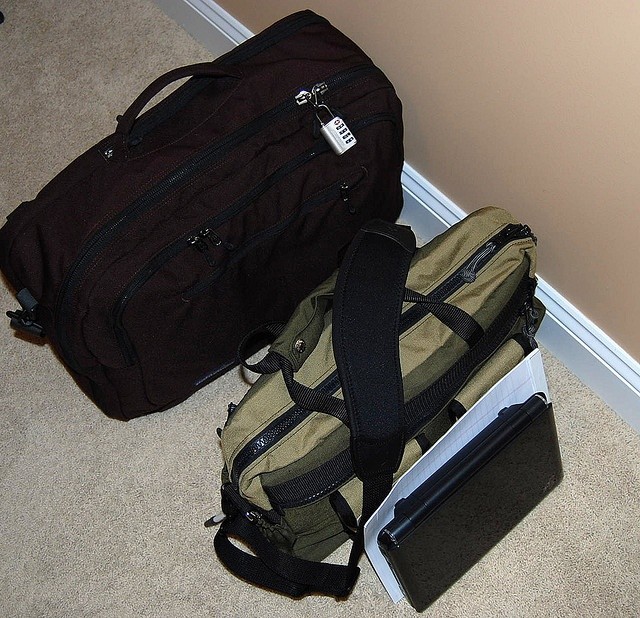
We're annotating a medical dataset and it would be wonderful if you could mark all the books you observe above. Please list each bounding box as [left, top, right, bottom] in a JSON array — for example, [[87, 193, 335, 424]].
[[362, 347, 553, 605]]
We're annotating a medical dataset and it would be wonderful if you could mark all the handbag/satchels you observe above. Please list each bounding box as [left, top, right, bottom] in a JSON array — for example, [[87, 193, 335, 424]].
[[203, 204, 547, 599], [0, 9, 404, 423]]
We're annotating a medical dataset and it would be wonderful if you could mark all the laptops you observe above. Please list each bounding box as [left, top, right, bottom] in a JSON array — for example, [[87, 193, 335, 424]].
[[378, 392, 564, 614]]
